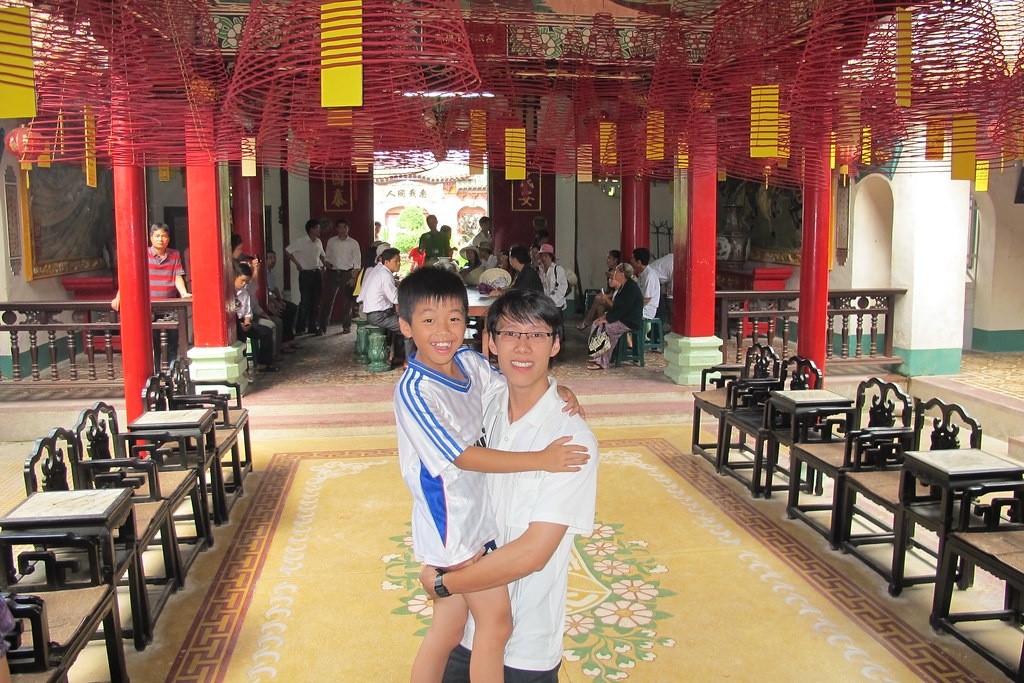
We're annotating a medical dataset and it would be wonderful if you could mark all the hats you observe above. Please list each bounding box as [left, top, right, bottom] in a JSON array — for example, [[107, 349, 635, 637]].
[[538, 243, 553, 254], [479, 268, 511, 289], [460, 245, 482, 261], [375, 244, 391, 264], [477, 241, 492, 251]]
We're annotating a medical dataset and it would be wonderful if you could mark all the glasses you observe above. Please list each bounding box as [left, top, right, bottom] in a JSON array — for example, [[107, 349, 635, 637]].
[[492, 328, 557, 343]]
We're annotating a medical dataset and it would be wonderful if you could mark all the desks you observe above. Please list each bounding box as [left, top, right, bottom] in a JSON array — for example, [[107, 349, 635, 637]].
[[126, 409, 220, 548], [897, 449, 1024, 631], [0, 487, 152, 683], [466, 283, 500, 359], [761, 388, 856, 517]]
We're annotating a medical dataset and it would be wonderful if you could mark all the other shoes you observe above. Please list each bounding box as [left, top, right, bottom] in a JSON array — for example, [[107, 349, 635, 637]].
[[271, 355, 284, 361], [315, 327, 327, 335], [343, 325, 350, 333], [402, 362, 408, 371], [289, 342, 303, 349], [575, 322, 588, 330], [586, 363, 603, 370], [279, 346, 296, 353], [391, 355, 405, 365], [257, 364, 279, 372], [295, 327, 304, 336], [308, 327, 318, 334]]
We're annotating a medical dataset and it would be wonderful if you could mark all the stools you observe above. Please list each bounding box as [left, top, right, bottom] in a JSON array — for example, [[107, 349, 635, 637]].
[[611, 324, 646, 367], [352, 318, 370, 364], [365, 326, 391, 372], [643, 318, 664, 354]]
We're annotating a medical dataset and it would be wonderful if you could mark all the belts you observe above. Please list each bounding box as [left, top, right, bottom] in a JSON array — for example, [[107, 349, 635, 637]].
[[304, 268, 323, 272]]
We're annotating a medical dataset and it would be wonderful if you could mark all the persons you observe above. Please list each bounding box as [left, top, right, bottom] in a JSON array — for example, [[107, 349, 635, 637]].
[[315, 221, 361, 335], [417, 287, 599, 683], [573, 247, 675, 370], [228, 230, 303, 382], [110, 222, 194, 374], [353, 214, 458, 371], [284, 217, 328, 334], [458, 216, 568, 340], [390, 265, 585, 683]]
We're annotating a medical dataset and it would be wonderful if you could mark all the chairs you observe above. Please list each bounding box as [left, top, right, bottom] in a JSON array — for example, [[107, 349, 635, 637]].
[[0, 356, 254, 683], [692, 343, 1024, 682]]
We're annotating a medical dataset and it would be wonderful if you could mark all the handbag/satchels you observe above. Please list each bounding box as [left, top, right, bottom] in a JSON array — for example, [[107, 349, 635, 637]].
[[588, 322, 611, 358], [554, 265, 572, 298]]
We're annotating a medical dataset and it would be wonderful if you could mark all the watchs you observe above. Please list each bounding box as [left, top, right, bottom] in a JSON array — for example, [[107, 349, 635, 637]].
[[434, 574, 452, 597]]
